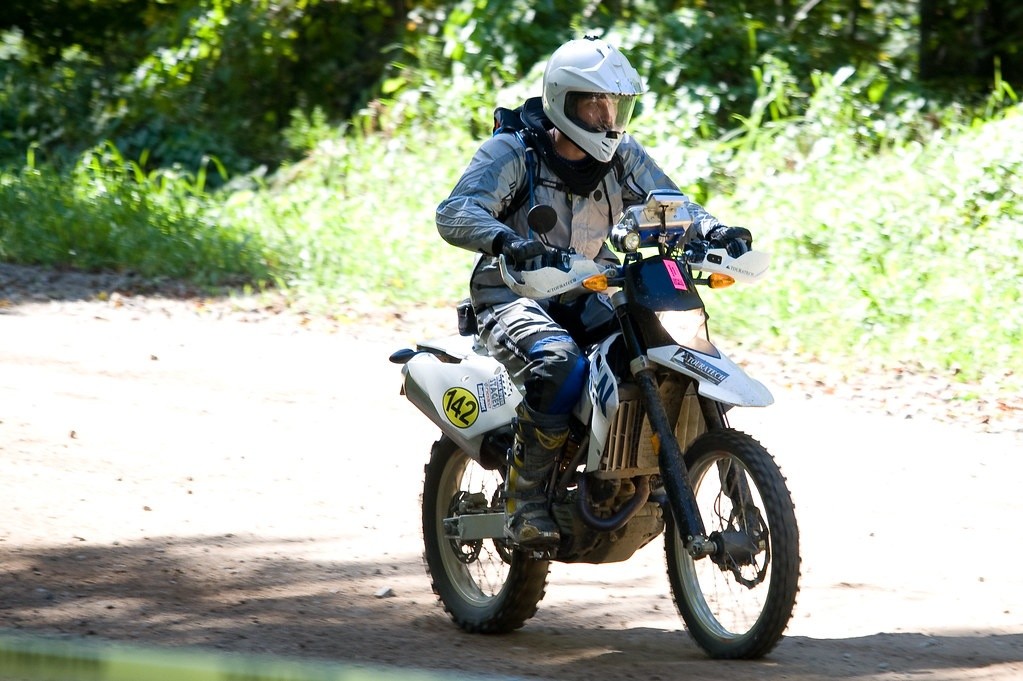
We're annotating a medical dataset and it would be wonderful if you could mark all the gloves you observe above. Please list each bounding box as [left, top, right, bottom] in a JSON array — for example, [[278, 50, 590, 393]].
[[497, 232, 548, 271], [704, 226, 752, 258]]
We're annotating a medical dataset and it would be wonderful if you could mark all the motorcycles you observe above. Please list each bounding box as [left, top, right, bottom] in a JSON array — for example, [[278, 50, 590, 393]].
[[389, 189, 803, 661]]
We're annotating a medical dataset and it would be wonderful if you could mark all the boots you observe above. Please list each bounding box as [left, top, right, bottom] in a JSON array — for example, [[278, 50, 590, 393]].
[[504, 395, 574, 547]]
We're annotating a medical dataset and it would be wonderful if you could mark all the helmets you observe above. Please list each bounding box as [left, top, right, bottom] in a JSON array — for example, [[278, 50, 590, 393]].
[[542, 36, 643, 163]]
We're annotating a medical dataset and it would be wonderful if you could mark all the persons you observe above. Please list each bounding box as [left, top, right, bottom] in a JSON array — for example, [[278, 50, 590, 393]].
[[436, 39, 752, 546]]
